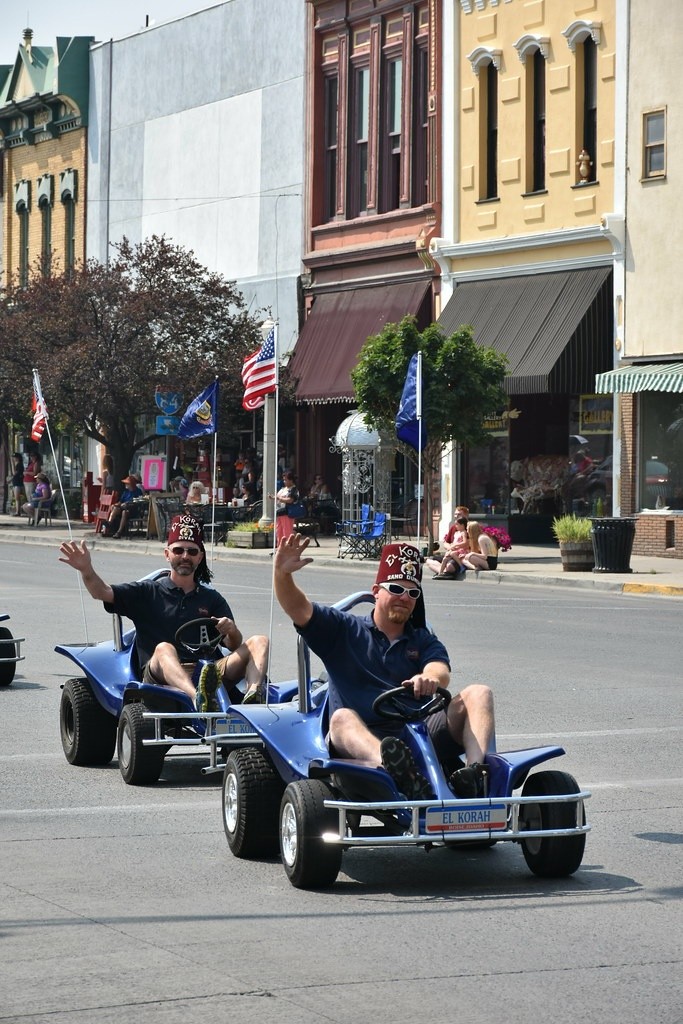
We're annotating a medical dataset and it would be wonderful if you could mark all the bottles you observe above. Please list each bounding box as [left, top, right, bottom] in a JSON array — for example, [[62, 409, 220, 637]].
[[233, 497, 237, 506]]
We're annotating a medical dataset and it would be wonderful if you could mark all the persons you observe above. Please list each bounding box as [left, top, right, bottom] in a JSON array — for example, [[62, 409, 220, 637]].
[[172, 448, 332, 518], [273, 533, 494, 814], [60, 515, 269, 728], [97, 454, 114, 494], [22, 473, 51, 526], [7, 452, 41, 518], [103, 473, 144, 538], [425, 506, 497, 577]]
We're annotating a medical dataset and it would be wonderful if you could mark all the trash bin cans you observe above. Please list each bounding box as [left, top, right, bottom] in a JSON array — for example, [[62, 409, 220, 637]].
[[587, 516, 640, 574]]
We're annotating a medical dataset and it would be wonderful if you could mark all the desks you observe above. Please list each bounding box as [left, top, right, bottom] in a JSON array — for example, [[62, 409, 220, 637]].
[[301, 497, 337, 535], [209, 505, 256, 543]]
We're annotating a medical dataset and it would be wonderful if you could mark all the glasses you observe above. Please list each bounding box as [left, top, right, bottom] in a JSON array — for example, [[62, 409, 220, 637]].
[[193, 486, 199, 490], [379, 582, 421, 599], [315, 478, 322, 480], [168, 547, 201, 556]]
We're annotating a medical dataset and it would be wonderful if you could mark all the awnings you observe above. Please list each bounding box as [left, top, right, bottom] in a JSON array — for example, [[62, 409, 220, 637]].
[[430, 265, 613, 396], [595, 361, 683, 394], [282, 281, 430, 406]]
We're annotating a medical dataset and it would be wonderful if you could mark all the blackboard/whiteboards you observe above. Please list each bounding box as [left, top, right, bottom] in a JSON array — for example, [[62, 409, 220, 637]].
[[147, 493, 186, 537]]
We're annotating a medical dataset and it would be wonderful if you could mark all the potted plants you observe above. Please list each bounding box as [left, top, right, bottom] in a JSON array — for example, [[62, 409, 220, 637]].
[[551, 512, 596, 572]]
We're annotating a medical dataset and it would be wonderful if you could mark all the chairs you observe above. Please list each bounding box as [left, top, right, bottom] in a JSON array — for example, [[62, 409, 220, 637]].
[[116, 503, 148, 540], [156, 500, 263, 543], [29, 489, 56, 527], [345, 508, 390, 560], [388, 496, 425, 541], [293, 500, 321, 547], [334, 503, 374, 559]]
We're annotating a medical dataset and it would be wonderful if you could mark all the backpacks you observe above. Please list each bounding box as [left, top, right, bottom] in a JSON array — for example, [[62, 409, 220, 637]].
[[482, 526, 513, 552]]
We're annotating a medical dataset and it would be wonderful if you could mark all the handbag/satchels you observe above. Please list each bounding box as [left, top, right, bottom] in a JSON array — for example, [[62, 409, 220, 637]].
[[286, 494, 308, 519]]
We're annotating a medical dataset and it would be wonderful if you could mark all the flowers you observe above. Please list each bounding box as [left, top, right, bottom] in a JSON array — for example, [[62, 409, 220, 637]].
[[483, 525, 512, 552]]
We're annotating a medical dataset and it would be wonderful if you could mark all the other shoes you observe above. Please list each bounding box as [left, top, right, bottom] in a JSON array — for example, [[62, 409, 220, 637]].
[[380, 735, 439, 813], [31, 516, 41, 526], [112, 531, 121, 539]]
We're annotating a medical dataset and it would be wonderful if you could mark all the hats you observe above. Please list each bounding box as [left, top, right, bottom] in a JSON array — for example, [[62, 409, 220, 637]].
[[167, 514, 203, 546], [376, 544, 422, 588], [34, 473, 46, 479], [121, 476, 142, 484], [11, 452, 21, 458]]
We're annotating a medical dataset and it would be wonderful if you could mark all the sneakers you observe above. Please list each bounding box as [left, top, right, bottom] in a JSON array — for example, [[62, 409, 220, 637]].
[[241, 691, 265, 705], [449, 764, 489, 798], [197, 663, 222, 722], [431, 572, 455, 580]]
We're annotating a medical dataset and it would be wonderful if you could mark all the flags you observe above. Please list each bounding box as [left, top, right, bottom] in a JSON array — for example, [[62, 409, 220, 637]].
[[395, 353, 427, 452], [176, 380, 218, 439], [240, 325, 276, 410], [29, 380, 49, 441]]
